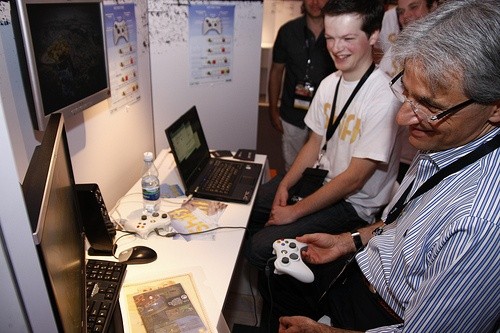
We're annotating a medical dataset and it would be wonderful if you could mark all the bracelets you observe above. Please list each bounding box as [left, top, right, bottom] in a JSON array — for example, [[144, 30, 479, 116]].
[[350, 229, 364, 253]]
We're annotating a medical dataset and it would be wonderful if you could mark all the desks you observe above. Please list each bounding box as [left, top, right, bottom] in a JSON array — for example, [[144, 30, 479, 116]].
[[85, 149, 267, 333]]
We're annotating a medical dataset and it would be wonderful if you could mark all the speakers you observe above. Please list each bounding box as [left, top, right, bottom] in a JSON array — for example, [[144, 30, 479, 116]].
[[75, 184, 117, 254]]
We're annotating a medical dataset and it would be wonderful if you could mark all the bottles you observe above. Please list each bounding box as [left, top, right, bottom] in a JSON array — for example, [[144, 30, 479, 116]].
[[141, 152, 160, 212]]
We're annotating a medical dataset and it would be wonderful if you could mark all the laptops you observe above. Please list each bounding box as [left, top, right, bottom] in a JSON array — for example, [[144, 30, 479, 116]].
[[164, 106, 262, 203]]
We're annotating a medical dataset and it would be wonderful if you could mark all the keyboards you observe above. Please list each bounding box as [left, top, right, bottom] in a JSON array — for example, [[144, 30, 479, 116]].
[[86, 260, 127, 333]]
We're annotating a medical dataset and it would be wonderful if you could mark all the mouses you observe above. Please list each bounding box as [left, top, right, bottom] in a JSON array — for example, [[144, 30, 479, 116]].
[[119, 245, 158, 265]]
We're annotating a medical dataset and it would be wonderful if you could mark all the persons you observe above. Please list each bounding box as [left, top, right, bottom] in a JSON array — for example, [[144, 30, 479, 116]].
[[258, 0, 500, 333], [268, 0, 340, 172], [380, 0, 444, 185], [243, 0, 399, 273]]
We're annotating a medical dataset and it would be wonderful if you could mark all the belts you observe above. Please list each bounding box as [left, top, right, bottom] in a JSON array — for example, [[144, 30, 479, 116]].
[[356, 267, 402, 322]]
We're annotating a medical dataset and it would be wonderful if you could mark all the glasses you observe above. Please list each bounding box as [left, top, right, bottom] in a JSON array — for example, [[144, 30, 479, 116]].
[[389, 69, 475, 122]]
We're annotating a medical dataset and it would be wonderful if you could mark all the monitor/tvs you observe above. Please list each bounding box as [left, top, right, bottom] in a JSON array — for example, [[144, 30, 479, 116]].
[[24, 114, 90, 333], [12, 0, 112, 130]]
